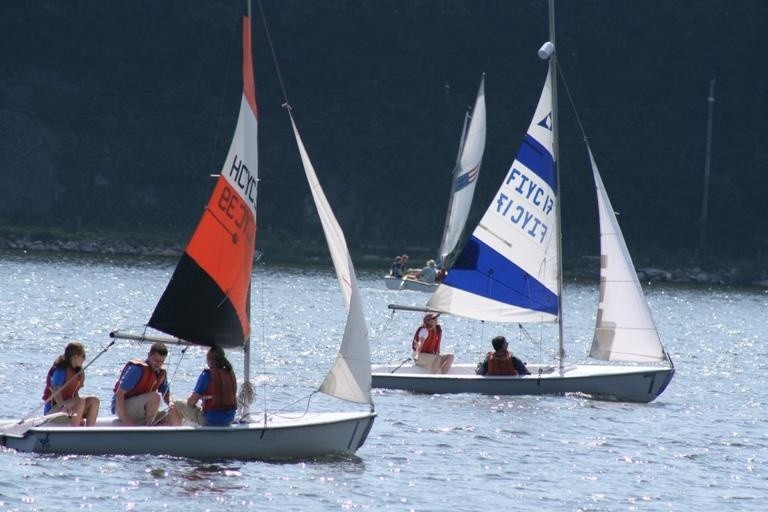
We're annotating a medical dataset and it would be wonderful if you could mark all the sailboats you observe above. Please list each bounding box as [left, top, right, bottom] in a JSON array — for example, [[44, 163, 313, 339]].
[[0, 0, 381, 465], [370, 0, 676, 406], [385, 69, 486, 295]]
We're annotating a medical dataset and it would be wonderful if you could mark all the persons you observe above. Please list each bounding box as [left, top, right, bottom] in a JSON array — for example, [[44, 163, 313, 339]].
[[411, 314, 454, 374], [475, 336, 530, 375], [168, 346, 237, 426], [42, 341, 100, 427], [111, 342, 170, 426], [392, 252, 447, 283]]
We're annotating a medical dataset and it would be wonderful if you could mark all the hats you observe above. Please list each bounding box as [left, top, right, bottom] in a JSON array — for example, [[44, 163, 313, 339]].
[[423, 313, 439, 323]]
